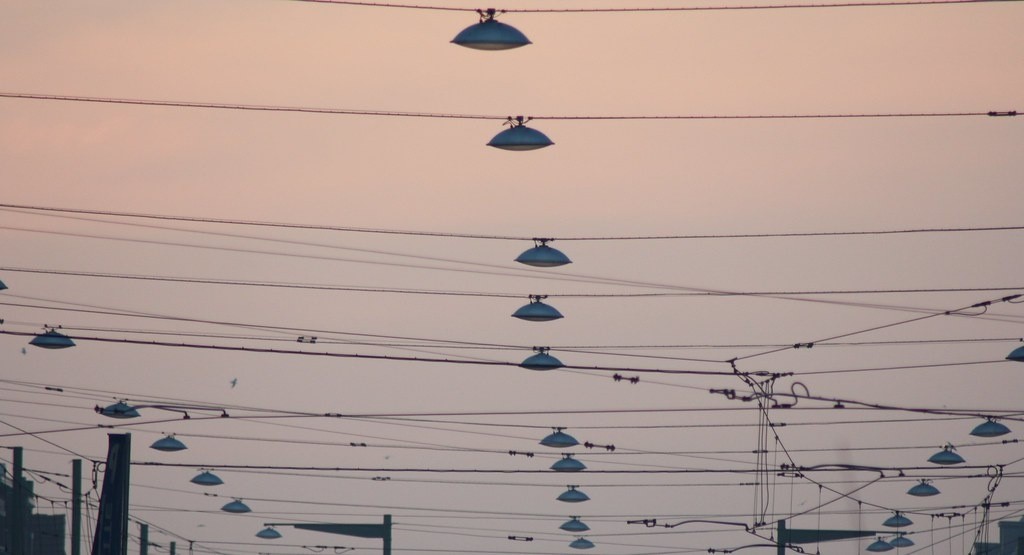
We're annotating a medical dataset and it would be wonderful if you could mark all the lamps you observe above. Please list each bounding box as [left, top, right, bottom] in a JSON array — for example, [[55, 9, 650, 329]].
[[150, 437, 282, 539], [1005, 346, 1024, 362], [865, 539, 892, 552], [538, 431, 580, 447], [906, 482, 939, 496], [510, 297, 564, 321], [555, 486, 591, 502], [559, 519, 589, 532], [927, 449, 965, 465], [486, 119, 554, 152], [968, 419, 1012, 438], [514, 237, 574, 268], [569, 539, 595, 549], [549, 454, 587, 471], [27, 332, 77, 349], [889, 536, 915, 547], [519, 349, 563, 371], [882, 515, 913, 527], [450, 11, 532, 52], [102, 401, 140, 419]]
